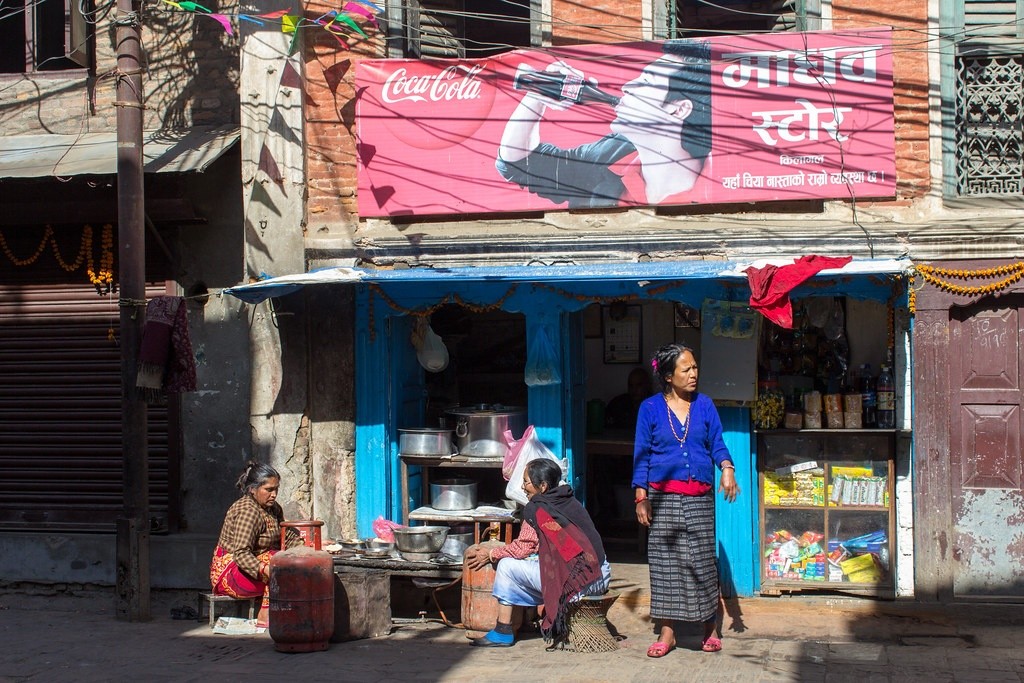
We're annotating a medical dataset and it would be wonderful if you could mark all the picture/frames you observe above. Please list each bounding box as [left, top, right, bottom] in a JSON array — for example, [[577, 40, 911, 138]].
[[583, 303, 602, 339], [600, 303, 642, 365]]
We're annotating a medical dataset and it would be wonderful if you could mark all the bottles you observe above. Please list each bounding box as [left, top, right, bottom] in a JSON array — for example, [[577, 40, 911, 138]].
[[513, 63, 622, 107], [858, 364, 895, 429]]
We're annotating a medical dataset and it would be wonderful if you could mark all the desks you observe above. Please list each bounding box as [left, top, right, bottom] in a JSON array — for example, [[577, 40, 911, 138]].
[[585, 439, 646, 555], [331, 554, 463, 639]]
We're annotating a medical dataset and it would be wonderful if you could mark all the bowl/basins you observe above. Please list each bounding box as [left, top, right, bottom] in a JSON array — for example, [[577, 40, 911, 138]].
[[393, 526, 451, 553], [429, 479, 480, 510], [397, 428, 454, 455], [338, 542, 365, 558], [364, 539, 395, 556], [439, 527, 475, 557]]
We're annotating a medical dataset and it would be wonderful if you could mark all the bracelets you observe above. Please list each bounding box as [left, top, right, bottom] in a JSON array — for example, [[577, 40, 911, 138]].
[[722, 465, 735, 472], [490, 549, 496, 562], [635, 497, 649, 503]]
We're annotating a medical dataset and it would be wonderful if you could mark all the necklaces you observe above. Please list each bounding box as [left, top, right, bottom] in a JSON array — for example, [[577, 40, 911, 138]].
[[667, 394, 690, 447]]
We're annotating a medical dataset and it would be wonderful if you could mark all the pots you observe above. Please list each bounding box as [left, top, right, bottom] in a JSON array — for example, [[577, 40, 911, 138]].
[[444, 406, 528, 457]]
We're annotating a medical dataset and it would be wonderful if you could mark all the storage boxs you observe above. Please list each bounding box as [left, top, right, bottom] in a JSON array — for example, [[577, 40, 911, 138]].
[[766, 459, 888, 584]]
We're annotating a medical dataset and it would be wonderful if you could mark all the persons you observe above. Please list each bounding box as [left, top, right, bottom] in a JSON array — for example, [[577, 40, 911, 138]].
[[210, 464, 303, 628], [632, 345, 741, 656], [467, 458, 611, 646], [495, 39, 713, 207]]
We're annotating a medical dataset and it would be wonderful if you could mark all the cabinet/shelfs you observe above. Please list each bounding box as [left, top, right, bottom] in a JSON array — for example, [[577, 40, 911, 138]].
[[398, 453, 520, 527], [759, 433, 894, 597]]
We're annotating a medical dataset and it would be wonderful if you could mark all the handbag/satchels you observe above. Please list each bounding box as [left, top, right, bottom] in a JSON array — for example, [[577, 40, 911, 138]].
[[505, 427, 569, 506], [502, 425, 535, 480]]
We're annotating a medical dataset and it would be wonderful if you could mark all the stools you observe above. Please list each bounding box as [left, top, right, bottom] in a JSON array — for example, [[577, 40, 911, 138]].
[[198, 592, 255, 627]]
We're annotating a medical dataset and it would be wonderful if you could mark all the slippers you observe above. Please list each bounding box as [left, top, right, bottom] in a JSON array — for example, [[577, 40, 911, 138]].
[[647, 642, 671, 657], [256, 623, 269, 627], [701, 637, 722, 651]]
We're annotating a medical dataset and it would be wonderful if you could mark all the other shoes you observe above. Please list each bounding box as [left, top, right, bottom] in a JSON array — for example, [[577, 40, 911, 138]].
[[469, 629, 514, 646]]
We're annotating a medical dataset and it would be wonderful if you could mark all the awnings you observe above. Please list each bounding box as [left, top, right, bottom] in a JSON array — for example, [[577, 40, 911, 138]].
[[223, 259, 914, 302], [0, 125, 239, 177]]
